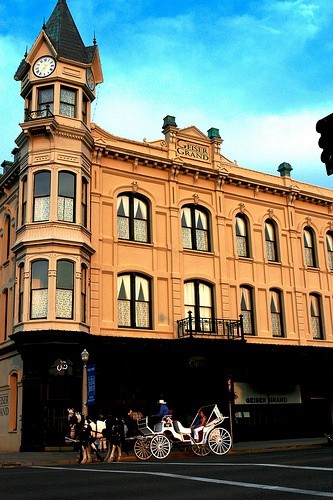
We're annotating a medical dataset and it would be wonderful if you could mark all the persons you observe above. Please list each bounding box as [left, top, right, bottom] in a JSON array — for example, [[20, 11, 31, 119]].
[[150, 400, 169, 423], [192, 412, 207, 430]]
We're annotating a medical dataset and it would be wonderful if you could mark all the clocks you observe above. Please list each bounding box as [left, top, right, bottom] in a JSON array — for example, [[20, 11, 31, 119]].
[[32, 56, 56, 78], [86, 67, 95, 91]]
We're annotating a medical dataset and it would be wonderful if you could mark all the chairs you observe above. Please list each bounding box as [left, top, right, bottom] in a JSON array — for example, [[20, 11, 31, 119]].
[[153, 410, 173, 431]]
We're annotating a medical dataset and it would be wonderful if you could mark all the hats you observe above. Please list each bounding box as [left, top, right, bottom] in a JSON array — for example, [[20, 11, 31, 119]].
[[158, 400, 166, 404]]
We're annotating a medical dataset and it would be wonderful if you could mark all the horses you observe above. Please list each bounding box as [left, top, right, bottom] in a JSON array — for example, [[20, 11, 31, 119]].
[[67, 407, 126, 464]]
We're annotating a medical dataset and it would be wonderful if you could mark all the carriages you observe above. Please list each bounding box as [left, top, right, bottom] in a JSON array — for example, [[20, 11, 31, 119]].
[[66, 404, 232, 467]]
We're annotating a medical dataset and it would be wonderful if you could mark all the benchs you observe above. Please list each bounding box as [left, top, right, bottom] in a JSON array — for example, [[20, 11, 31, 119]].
[[174, 420, 192, 434]]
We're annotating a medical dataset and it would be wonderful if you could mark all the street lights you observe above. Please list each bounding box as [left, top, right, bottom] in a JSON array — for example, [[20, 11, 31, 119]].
[[81, 349, 90, 420]]
